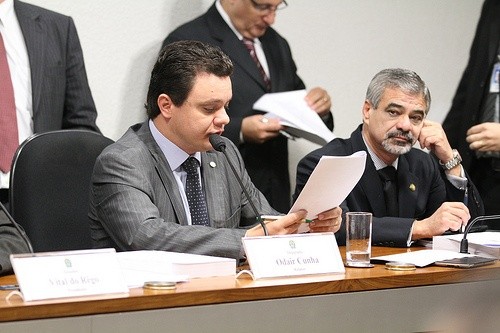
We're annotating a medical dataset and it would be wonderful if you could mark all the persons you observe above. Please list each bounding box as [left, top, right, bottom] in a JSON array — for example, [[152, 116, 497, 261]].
[[0, 0, 102, 210], [147, 0, 335, 215], [87, 41, 343, 267], [291, 67, 484, 248], [430, 0, 500, 230], [0, 209, 30, 276]]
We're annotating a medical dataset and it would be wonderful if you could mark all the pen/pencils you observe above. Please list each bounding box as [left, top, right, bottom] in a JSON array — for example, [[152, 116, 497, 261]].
[[255, 214, 315, 223], [260, 118, 295, 141]]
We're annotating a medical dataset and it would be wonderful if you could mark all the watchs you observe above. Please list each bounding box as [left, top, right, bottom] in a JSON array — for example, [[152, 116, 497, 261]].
[[439, 149, 462, 170]]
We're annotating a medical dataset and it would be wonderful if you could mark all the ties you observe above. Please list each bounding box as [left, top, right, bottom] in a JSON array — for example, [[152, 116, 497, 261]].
[[181, 157, 208, 226], [240, 37, 270, 92], [379, 166, 398, 217], [0, 32, 19, 173]]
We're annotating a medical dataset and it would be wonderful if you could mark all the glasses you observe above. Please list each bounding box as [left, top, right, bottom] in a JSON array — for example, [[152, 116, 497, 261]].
[[251, 0, 288, 11]]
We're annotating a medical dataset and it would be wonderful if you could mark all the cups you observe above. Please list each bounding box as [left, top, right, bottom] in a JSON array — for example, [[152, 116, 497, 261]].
[[346, 212, 372, 267]]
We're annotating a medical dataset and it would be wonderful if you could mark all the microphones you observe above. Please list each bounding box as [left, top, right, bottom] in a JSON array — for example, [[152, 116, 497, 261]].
[[209, 133, 269, 266]]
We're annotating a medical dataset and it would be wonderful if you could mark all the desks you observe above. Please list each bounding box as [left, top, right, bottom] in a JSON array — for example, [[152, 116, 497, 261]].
[[0, 244, 500, 333]]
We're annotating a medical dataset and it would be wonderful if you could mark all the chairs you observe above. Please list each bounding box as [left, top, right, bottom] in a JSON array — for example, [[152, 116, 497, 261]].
[[8, 130, 114, 252]]
